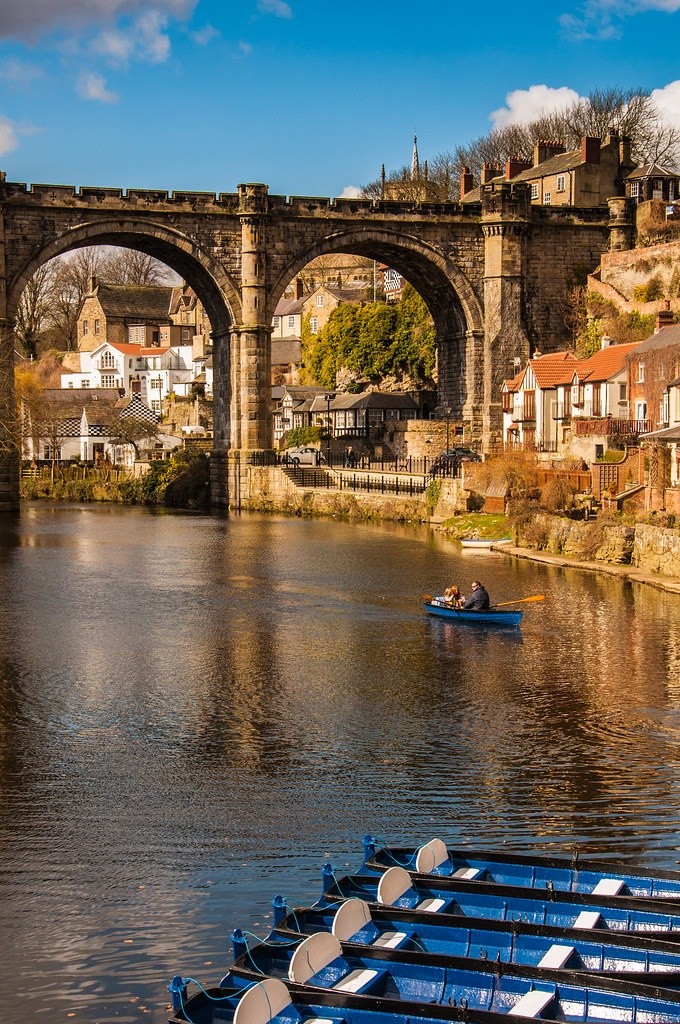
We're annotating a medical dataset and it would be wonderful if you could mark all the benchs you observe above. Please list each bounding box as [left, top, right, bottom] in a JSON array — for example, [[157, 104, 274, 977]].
[[389, 460, 409, 472], [537, 944, 575, 970], [288, 932, 388, 994], [591, 878, 624, 896], [508, 990, 554, 1019], [354, 457, 370, 469], [377, 866, 455, 913], [415, 837, 488, 879], [233, 978, 345, 1023], [573, 910, 600, 930], [332, 898, 415, 949]]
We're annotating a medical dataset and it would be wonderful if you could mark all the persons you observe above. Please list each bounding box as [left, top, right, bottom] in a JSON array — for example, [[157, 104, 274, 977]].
[[344, 444, 357, 469], [444, 585, 465, 607], [463, 581, 489, 611]]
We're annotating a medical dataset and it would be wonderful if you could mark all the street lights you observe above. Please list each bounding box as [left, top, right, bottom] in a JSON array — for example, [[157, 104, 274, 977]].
[[444, 406, 453, 475]]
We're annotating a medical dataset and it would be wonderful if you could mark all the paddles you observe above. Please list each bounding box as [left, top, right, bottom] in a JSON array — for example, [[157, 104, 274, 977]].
[[489, 595, 546, 608], [421, 593, 459, 608]]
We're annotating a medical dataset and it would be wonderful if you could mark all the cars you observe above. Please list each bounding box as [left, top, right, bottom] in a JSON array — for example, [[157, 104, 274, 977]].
[[282, 447, 325, 466], [436, 447, 483, 467]]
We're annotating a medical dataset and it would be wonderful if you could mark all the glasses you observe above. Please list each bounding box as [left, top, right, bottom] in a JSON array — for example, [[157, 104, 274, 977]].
[[472, 585, 478, 588]]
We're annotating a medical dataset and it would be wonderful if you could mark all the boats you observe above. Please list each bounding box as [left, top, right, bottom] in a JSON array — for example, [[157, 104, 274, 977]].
[[311, 862, 680, 944], [423, 596, 523, 625], [353, 834, 680, 903], [217, 928, 680, 1024], [167, 974, 470, 1024], [262, 894, 680, 991]]
